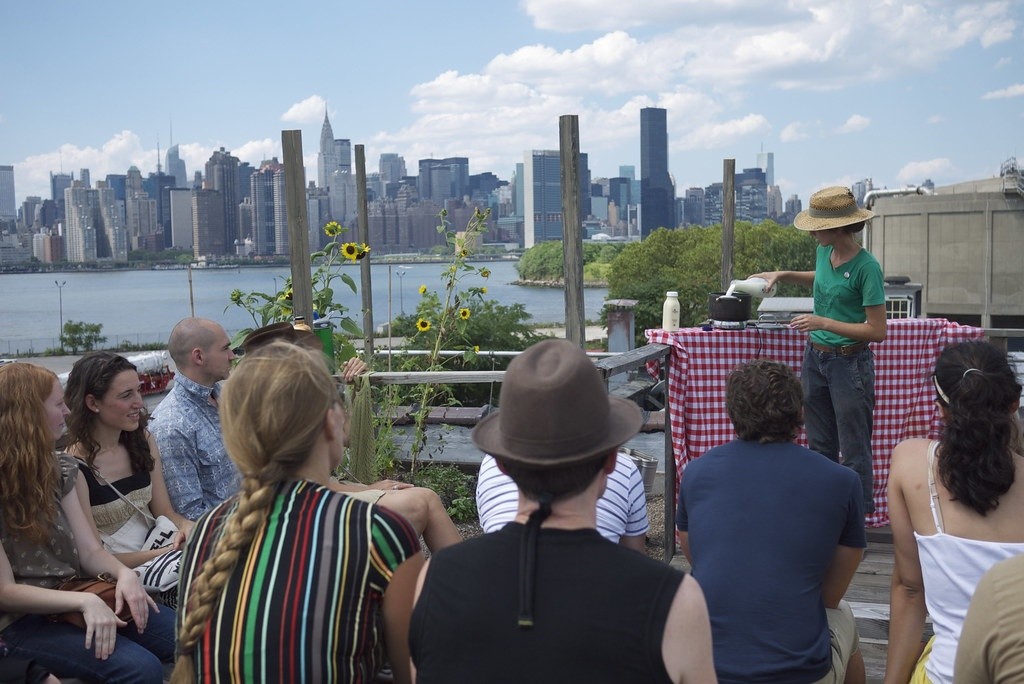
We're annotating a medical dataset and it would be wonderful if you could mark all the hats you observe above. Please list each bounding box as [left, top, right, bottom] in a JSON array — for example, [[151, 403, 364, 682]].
[[235, 321, 322, 369], [794, 186, 875, 230], [471, 339, 643, 468]]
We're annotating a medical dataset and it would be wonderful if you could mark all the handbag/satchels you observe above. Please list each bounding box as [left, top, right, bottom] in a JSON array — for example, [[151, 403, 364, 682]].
[[57, 572, 133, 629], [103, 511, 185, 593]]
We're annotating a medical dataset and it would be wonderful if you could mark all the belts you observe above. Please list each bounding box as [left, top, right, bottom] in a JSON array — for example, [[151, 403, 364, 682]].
[[806, 335, 867, 355]]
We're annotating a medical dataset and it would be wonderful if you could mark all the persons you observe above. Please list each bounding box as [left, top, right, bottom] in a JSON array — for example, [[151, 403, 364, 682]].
[[408, 338, 718, 684], [953, 555, 1024, 684], [476, 450, 650, 552], [676, 358, 868, 684], [169, 341, 426, 684], [0, 361, 177, 684], [748, 186, 888, 515], [243, 322, 463, 556], [884, 341, 1024, 684], [62, 351, 196, 608], [149, 317, 244, 523]]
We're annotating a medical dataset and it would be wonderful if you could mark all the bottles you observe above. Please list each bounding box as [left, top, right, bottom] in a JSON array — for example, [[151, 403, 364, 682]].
[[730, 277, 777, 298], [663, 291, 680, 332]]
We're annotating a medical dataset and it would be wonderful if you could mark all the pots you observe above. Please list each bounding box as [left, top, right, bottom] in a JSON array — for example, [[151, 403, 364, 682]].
[[708, 291, 752, 321]]
